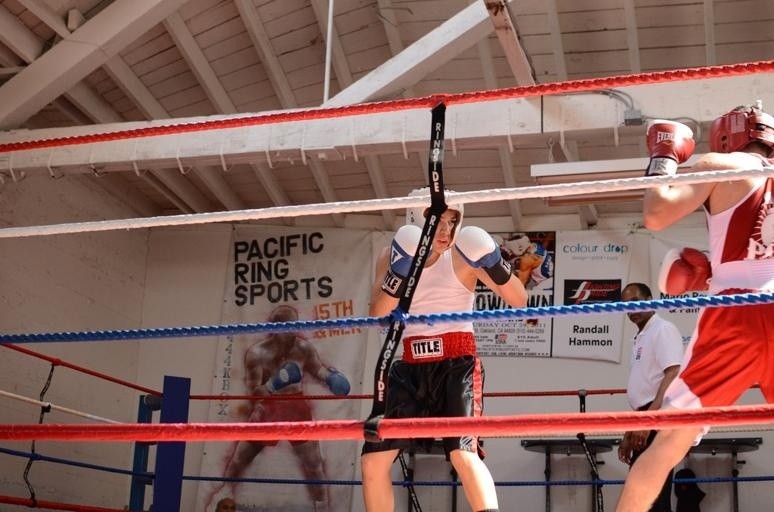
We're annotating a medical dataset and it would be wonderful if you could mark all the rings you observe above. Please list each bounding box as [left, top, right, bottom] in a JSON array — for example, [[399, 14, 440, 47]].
[[637, 441, 643, 445]]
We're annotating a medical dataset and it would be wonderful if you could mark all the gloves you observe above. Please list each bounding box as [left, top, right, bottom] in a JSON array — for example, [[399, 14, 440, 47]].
[[379, 225, 433, 298], [455, 225, 512, 286], [643, 119, 696, 176], [657, 246, 713, 297], [325, 366, 350, 396], [265, 362, 302, 394]]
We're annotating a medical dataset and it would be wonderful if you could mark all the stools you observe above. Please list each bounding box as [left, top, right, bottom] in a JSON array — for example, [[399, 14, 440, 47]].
[[686, 435, 765, 512], [520, 434, 625, 512], [399, 437, 484, 512]]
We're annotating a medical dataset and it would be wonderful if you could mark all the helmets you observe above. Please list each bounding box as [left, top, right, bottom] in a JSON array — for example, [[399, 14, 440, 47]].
[[709, 105, 774, 167], [506, 236, 532, 256], [406, 186, 464, 254]]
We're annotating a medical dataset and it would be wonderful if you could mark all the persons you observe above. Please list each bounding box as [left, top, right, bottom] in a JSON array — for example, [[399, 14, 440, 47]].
[[214, 498, 236, 512], [355, 183, 530, 512], [190, 302, 352, 512], [614, 281, 713, 512], [611, 99, 773, 512], [498, 229, 553, 294], [671, 467, 708, 512]]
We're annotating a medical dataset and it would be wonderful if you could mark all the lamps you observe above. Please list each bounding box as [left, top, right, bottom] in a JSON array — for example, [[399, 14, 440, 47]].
[[530, 135, 710, 206]]
